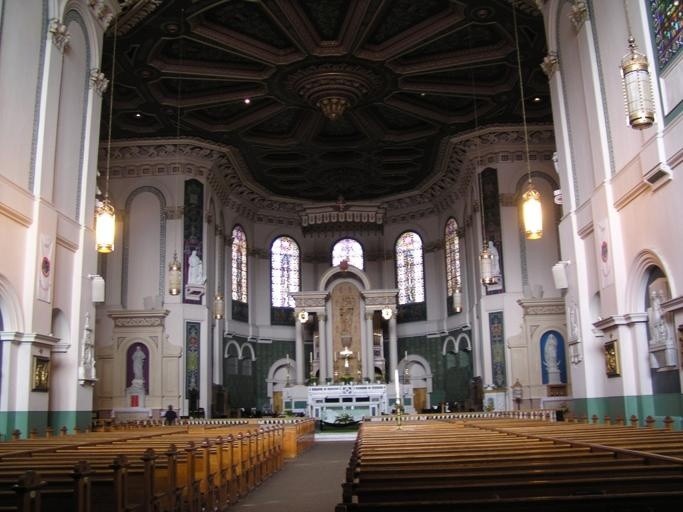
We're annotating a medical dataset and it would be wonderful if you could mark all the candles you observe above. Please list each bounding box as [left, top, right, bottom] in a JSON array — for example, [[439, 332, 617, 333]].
[[395, 369, 400, 404]]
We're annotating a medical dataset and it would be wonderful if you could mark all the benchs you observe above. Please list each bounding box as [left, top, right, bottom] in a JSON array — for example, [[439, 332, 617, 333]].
[[0, 416, 316, 512], [335, 412, 683, 512]]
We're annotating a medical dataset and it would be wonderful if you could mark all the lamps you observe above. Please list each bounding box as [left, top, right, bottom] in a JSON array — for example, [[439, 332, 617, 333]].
[[618, 1, 655, 131], [511, 378, 522, 402], [468, 1, 500, 286], [169, 0, 182, 295], [213, 286, 225, 319], [95, 0, 115, 253], [452, 284, 463, 312], [512, 0, 543, 240]]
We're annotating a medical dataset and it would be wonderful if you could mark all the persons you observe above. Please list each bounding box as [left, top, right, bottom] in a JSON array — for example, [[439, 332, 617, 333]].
[[542, 333, 559, 374], [161, 404, 179, 426], [188, 250, 203, 284], [487, 241, 500, 275], [132, 343, 146, 381]]
